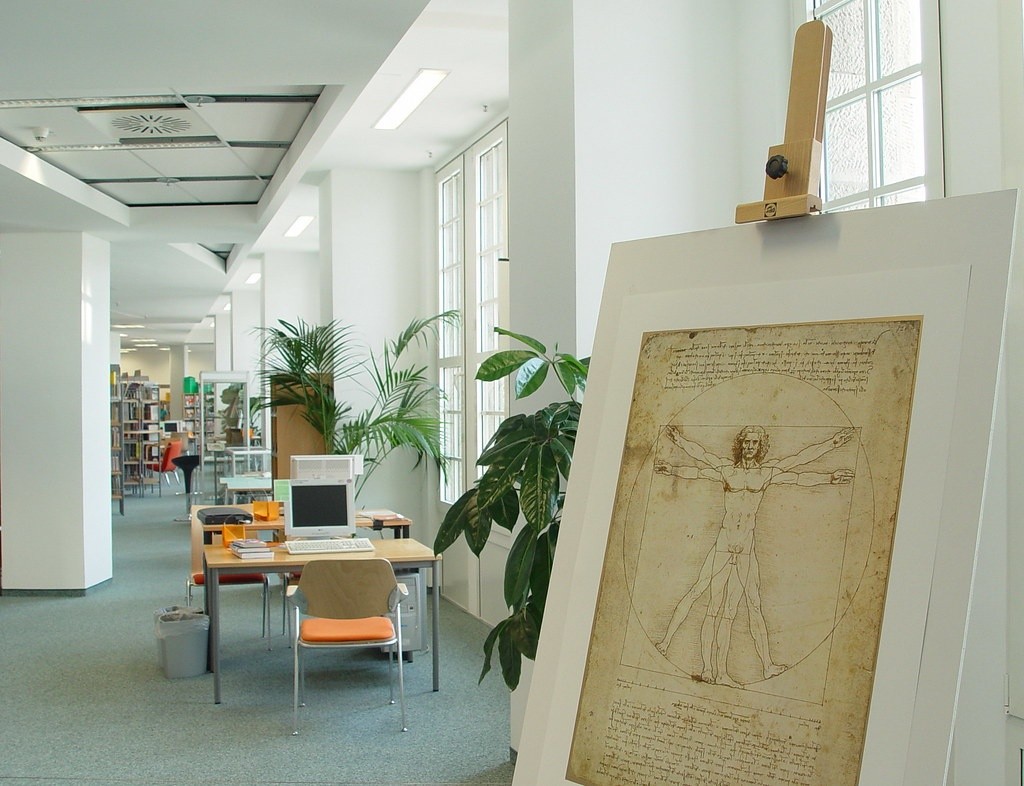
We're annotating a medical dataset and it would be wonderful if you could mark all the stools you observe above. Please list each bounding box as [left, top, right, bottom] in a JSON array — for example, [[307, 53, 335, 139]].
[[171, 454, 202, 522]]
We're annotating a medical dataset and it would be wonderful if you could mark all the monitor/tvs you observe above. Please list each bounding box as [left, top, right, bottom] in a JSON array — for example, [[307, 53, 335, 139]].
[[289, 455, 355, 480], [164, 421, 179, 435], [285, 480, 356, 541]]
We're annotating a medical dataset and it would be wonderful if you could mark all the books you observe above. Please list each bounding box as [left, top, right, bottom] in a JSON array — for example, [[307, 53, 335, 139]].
[[184, 396, 215, 456], [110, 369, 159, 496], [229, 539, 274, 559]]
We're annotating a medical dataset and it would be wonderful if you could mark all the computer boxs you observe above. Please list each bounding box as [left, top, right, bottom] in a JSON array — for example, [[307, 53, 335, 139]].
[[380, 572, 421, 653]]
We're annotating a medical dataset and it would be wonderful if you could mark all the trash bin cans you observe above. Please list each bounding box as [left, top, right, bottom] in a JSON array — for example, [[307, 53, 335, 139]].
[[154, 605, 210, 680]]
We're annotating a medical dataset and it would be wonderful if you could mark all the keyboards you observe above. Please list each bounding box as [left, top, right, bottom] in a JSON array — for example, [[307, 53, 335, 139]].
[[285, 537, 375, 555]]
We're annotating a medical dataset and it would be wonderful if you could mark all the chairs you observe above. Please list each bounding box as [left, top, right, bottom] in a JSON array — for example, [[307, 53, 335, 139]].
[[146, 441, 182, 488], [287, 558, 409, 733], [186, 504, 273, 652], [171, 431, 190, 456]]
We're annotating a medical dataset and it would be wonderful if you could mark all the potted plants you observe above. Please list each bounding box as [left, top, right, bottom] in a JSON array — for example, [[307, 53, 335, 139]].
[[432, 325, 591, 766]]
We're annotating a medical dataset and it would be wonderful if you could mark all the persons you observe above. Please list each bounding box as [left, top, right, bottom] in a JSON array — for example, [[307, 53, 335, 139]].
[[160, 405, 167, 421]]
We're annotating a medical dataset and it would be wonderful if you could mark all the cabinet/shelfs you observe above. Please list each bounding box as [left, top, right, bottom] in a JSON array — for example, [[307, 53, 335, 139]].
[[109, 363, 125, 516], [183, 392, 213, 455], [269, 372, 334, 490], [122, 382, 162, 499]]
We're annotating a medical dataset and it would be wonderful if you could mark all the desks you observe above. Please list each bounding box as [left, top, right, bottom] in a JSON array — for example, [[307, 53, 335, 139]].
[[201, 538, 443, 705], [193, 516, 412, 574], [214, 436, 261, 443], [227, 479, 272, 504], [218, 477, 271, 505]]
